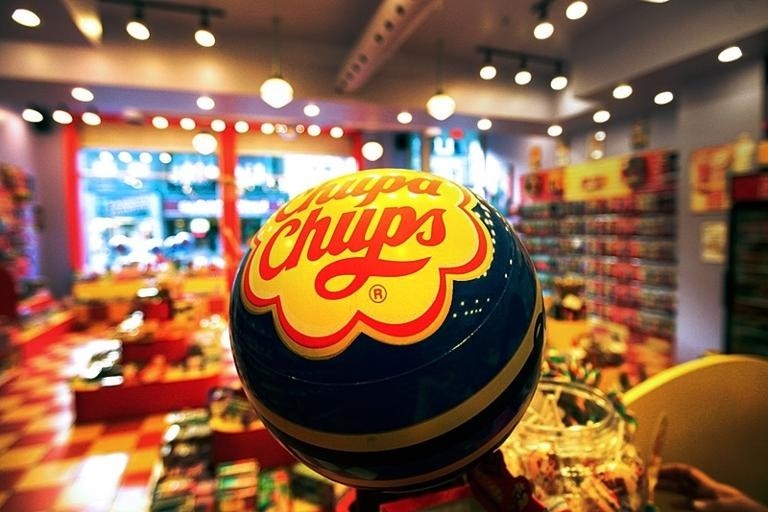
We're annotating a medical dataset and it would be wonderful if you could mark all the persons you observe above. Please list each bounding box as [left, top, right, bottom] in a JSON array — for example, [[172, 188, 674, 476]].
[[650, 462, 768, 510]]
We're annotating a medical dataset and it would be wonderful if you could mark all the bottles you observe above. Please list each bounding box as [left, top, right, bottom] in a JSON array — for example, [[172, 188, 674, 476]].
[[499, 380, 646, 511]]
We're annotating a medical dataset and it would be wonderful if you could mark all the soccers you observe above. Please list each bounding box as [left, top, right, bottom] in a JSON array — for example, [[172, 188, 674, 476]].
[[229, 168, 547, 488]]
